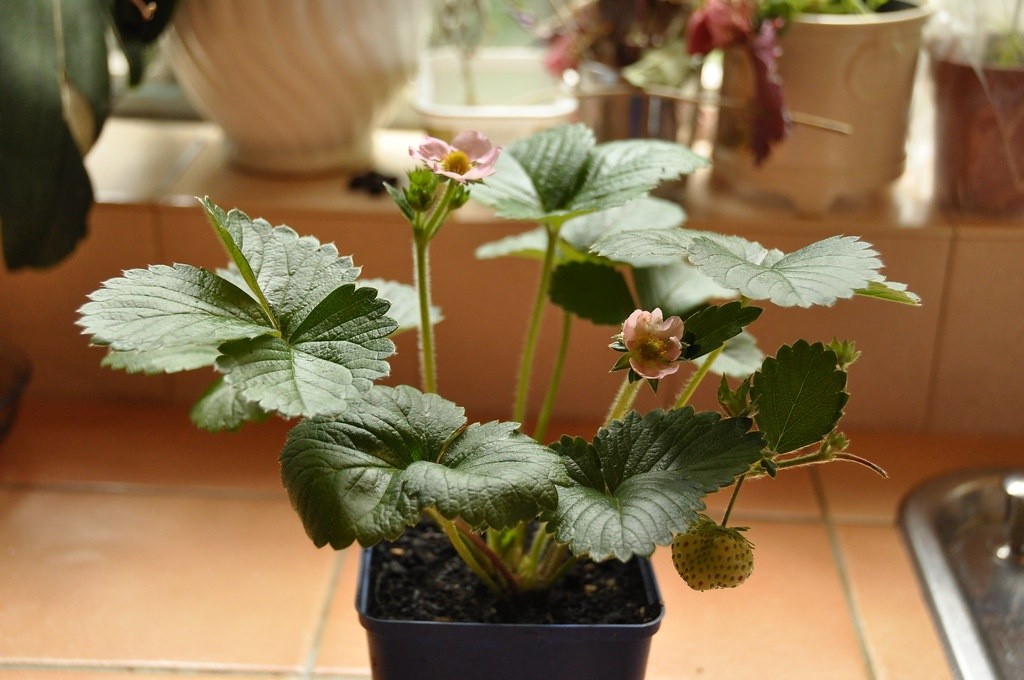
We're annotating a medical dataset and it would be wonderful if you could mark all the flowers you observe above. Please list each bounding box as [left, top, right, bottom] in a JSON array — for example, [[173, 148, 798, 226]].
[[71, 119, 921, 602], [528, 0, 902, 170]]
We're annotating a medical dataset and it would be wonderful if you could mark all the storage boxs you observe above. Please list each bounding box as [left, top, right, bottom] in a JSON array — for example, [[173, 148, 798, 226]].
[[416, 47, 580, 141]]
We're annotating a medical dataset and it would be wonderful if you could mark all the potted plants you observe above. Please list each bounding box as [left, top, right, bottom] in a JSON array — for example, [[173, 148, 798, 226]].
[[923, 1, 1023, 219], [107, 0, 431, 177]]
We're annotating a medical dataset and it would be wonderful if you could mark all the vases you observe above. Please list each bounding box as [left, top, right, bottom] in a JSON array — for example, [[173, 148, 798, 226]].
[[712, 0, 934, 217], [354, 531, 666, 680]]
